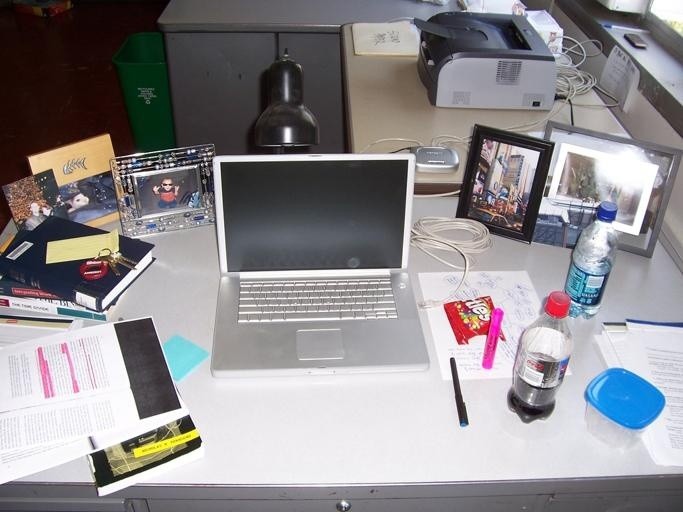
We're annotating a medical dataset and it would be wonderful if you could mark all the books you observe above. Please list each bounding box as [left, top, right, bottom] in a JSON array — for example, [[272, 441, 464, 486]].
[[0, 217, 156, 328], [88, 415, 204, 497]]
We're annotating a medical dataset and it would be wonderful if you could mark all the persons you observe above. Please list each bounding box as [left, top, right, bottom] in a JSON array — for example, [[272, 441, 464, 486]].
[[40, 204, 61, 217], [25, 202, 48, 229], [152, 179, 181, 210]]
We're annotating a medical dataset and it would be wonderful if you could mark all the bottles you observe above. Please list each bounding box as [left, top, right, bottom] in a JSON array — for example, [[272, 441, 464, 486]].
[[563, 202, 618, 318], [507, 293, 572, 423]]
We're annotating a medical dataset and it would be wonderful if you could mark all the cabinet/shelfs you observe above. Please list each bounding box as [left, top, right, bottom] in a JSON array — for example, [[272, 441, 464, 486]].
[[157, 1, 461, 154]]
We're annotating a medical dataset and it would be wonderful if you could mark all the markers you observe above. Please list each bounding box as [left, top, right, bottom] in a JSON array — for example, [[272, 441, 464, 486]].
[[482, 309, 503, 369]]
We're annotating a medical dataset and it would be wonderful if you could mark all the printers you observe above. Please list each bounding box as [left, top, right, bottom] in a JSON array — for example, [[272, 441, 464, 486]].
[[418, 11, 557, 111]]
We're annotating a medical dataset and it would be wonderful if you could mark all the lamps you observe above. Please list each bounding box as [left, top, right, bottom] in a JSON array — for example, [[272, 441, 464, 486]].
[[253, 49, 320, 156]]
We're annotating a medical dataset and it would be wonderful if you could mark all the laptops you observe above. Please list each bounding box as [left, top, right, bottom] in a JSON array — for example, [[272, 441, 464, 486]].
[[212, 154, 432, 376]]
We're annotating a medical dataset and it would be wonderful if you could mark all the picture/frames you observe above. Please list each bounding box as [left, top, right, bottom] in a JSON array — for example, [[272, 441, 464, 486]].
[[109, 144, 216, 239], [532, 119, 682, 259], [456, 123, 556, 243]]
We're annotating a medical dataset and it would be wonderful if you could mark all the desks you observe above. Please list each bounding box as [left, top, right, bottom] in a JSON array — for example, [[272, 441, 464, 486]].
[[342, 17, 661, 190], [0, 193, 682, 512]]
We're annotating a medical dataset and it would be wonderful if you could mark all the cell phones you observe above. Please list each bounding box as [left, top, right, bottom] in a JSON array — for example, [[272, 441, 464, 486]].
[[625, 32, 648, 49]]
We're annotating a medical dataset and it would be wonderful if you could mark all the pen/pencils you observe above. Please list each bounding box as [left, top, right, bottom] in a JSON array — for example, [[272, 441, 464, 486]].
[[450, 358, 469, 426]]
